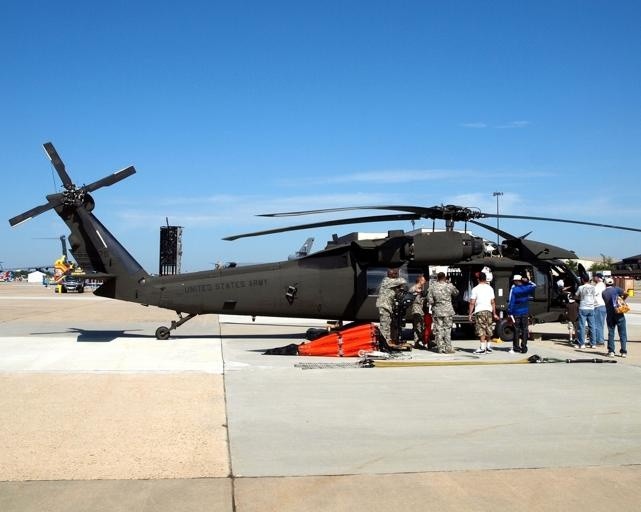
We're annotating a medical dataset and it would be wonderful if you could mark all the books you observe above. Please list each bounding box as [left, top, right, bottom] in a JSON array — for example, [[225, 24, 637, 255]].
[[508, 315, 517, 324]]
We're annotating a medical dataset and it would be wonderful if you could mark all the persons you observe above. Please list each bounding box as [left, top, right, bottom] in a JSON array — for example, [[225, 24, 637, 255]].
[[377, 269, 408, 345], [576, 273, 631, 358], [468, 273, 500, 354], [428, 272, 461, 353], [508, 274, 538, 353], [423, 295, 437, 346], [409, 276, 428, 348]]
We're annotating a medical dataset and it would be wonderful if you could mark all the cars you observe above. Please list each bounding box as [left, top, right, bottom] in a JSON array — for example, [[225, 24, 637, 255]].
[[60, 275, 83, 293]]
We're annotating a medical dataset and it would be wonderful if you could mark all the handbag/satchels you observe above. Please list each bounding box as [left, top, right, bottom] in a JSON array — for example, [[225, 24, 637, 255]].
[[612, 294, 629, 313]]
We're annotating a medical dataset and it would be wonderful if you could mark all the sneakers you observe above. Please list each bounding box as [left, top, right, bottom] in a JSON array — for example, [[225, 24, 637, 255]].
[[473, 347, 492, 354], [607, 352, 625, 358], [579, 342, 604, 349]]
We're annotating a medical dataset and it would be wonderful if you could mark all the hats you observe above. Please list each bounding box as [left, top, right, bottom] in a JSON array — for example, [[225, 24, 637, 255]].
[[513, 275, 522, 280], [606, 278, 614, 285]]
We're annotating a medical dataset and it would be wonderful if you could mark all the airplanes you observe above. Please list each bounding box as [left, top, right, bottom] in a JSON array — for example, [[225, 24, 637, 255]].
[[9, 140, 641, 342], [30, 236, 102, 285]]
